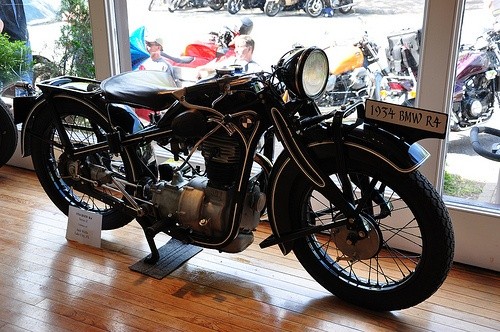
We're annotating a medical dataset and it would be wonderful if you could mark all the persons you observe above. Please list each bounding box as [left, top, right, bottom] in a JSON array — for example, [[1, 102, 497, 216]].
[[230, 35, 259, 72], [137, 35, 174, 77]]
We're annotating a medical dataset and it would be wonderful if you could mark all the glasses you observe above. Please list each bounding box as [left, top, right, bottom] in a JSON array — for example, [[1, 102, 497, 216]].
[[148, 43, 159, 48]]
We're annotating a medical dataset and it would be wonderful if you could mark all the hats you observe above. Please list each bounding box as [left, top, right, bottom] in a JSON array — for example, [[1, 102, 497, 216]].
[[146, 35, 166, 51]]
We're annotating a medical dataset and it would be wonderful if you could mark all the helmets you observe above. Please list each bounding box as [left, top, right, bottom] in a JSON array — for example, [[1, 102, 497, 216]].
[[234, 16, 253, 36]]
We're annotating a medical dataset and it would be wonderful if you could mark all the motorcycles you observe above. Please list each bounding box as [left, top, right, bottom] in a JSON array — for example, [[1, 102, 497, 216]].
[[146, 0, 360, 18], [13, 44, 455, 312], [312, 28, 500, 135]]
